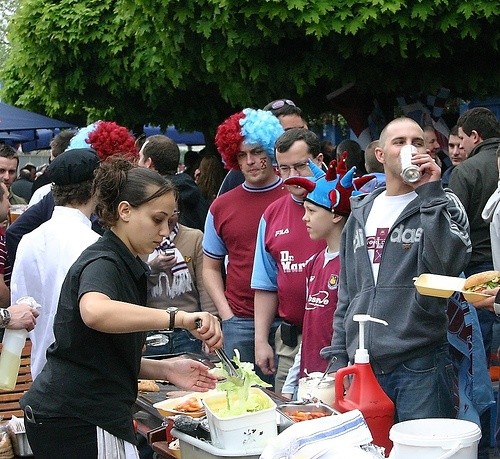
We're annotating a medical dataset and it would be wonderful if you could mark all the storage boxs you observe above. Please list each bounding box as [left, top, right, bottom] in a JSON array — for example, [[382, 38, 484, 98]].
[[412, 272, 492, 304], [201, 388, 279, 450]]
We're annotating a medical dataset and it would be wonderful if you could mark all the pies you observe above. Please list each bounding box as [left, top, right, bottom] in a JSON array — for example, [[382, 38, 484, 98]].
[[137, 379, 160, 391], [0, 435, 14, 459], [172, 396, 203, 412]]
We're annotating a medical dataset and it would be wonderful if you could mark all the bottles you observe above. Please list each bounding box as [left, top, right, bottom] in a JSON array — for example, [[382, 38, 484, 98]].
[[297, 376, 336, 407]]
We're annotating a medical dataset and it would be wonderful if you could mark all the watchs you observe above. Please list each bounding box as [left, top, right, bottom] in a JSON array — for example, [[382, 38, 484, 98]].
[[0, 308, 11, 328], [166, 306, 179, 331]]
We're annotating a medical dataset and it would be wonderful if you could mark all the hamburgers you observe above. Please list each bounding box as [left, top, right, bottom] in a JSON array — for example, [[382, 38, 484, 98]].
[[464, 270, 500, 296]]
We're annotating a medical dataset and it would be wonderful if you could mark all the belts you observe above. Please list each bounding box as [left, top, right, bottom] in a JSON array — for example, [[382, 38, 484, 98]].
[[150, 328, 182, 334]]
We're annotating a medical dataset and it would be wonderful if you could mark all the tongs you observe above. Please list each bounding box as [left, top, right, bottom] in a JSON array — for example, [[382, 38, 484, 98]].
[[251, 384, 291, 406], [195, 318, 239, 379]]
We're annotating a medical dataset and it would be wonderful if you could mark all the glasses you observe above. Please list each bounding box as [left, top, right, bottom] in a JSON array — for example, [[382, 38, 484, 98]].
[[275, 158, 308, 175], [267, 100, 298, 111]]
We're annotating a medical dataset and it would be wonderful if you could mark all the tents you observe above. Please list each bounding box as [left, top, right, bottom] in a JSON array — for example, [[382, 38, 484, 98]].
[[0, 101, 77, 152], [143, 124, 206, 146]]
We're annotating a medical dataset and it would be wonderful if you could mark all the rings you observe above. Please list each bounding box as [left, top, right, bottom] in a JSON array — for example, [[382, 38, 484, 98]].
[[195, 381, 200, 386], [432, 159, 435, 162]]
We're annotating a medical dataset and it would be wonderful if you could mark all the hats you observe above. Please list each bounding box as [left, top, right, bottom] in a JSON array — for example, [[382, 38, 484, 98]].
[[283, 151, 377, 218], [45, 148, 97, 186]]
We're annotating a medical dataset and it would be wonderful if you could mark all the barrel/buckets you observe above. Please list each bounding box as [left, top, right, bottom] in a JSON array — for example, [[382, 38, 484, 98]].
[[387, 417, 482, 459]]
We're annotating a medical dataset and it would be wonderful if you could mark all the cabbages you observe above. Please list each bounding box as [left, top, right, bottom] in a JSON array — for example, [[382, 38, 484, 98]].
[[213, 348, 273, 418]]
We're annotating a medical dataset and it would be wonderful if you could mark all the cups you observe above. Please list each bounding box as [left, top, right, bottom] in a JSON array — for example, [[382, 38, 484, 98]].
[[165, 249, 175, 262]]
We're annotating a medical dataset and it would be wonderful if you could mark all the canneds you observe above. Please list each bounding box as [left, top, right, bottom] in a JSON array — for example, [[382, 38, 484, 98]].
[[400, 144, 421, 183]]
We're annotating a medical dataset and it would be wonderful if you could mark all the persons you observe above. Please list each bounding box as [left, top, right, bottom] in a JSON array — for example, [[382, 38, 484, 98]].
[[0, 121, 230, 383], [201, 98, 500, 424], [17, 160, 224, 459]]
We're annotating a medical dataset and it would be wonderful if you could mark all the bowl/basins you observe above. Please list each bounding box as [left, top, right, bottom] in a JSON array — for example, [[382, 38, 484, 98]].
[[201, 387, 278, 450], [412, 273, 493, 303], [152, 393, 206, 419]]
